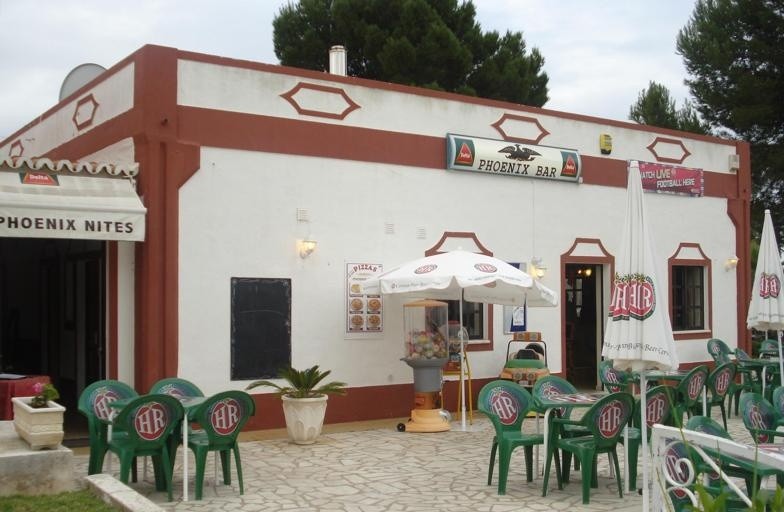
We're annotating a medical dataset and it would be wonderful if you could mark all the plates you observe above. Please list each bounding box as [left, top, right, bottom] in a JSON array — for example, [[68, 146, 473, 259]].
[[0, 373, 27, 380]]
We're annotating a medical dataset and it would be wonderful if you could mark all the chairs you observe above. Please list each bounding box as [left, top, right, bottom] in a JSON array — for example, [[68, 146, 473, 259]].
[[542, 390, 627, 503], [529, 373, 596, 473], [597, 339, 784, 512], [74, 376, 143, 486], [473, 378, 563, 492], [167, 388, 257, 495], [147, 377, 213, 484], [102, 392, 176, 502]]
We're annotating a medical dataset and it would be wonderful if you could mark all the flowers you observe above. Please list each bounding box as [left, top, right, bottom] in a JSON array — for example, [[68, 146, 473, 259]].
[[26, 379, 59, 406]]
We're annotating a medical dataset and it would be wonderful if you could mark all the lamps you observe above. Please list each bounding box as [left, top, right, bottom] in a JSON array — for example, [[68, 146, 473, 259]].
[[298, 238, 318, 259], [535, 267, 547, 280], [724, 253, 740, 272]]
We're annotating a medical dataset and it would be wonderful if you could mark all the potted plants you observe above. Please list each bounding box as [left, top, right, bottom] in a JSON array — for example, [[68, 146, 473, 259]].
[[241, 364, 350, 446]]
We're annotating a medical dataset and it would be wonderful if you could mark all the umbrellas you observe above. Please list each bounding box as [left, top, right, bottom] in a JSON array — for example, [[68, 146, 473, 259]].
[[746, 209, 784, 387], [602, 161, 680, 511], [362, 251, 559, 431]]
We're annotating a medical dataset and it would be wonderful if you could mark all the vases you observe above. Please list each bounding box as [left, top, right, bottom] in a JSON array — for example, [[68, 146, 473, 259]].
[[14, 396, 66, 449]]
